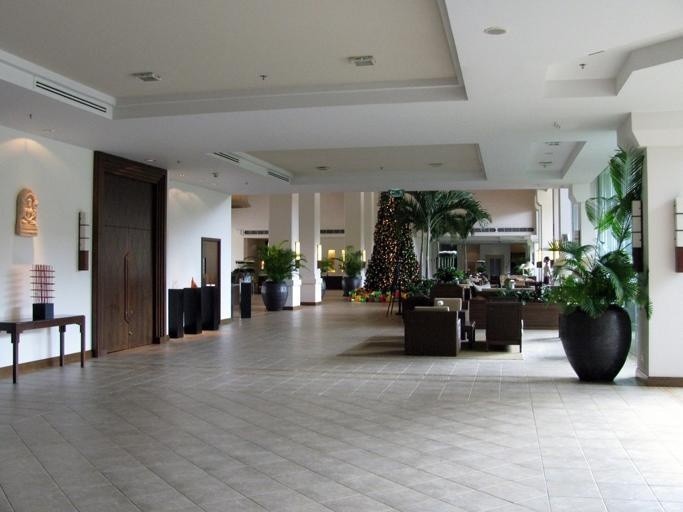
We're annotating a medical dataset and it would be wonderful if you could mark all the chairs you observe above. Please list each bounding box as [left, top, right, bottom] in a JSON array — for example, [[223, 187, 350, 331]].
[[401, 280, 526, 354]]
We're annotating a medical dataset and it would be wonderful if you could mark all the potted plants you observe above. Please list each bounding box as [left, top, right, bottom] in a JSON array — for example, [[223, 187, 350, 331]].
[[317, 260, 331, 300], [331, 245, 366, 296], [244, 241, 311, 312], [539, 145, 652, 388]]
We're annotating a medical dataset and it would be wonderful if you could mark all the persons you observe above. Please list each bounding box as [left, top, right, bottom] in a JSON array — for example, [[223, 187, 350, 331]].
[[21, 198, 36, 225], [542, 256, 552, 284]]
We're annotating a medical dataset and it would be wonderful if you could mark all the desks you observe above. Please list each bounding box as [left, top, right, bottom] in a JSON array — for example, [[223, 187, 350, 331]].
[[0, 315, 89, 384]]
